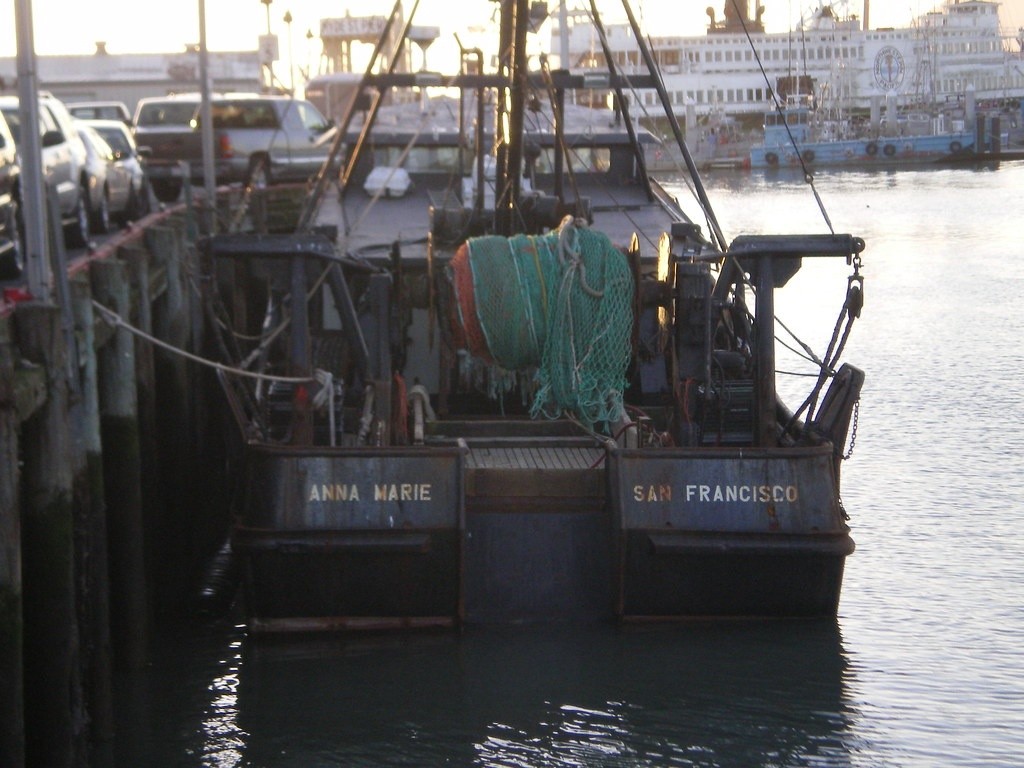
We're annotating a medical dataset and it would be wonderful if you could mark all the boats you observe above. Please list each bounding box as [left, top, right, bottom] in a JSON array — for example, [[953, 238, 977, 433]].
[[748, 0, 976, 170], [202, 0, 868, 644]]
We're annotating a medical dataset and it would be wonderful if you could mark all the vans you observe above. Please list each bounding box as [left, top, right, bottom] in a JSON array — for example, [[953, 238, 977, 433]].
[[0, 89, 352, 282]]
